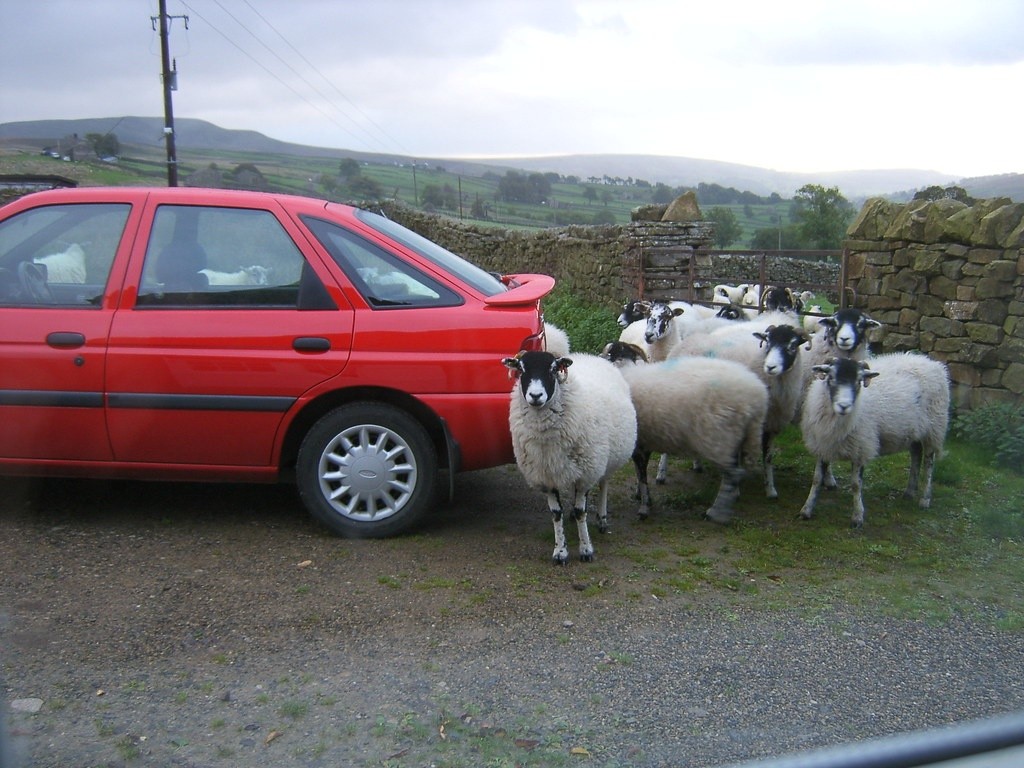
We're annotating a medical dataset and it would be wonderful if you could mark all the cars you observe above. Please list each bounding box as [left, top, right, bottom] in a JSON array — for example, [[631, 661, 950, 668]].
[[1, 188, 558, 537]]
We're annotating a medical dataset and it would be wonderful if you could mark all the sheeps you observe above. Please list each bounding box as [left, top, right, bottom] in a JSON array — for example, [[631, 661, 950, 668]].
[[31, 239, 93, 285], [501, 282, 950, 568], [355, 266, 442, 299], [197, 264, 274, 286]]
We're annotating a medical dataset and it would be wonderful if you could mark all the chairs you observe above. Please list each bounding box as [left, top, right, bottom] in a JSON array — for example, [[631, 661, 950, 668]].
[[155, 237, 209, 294]]
